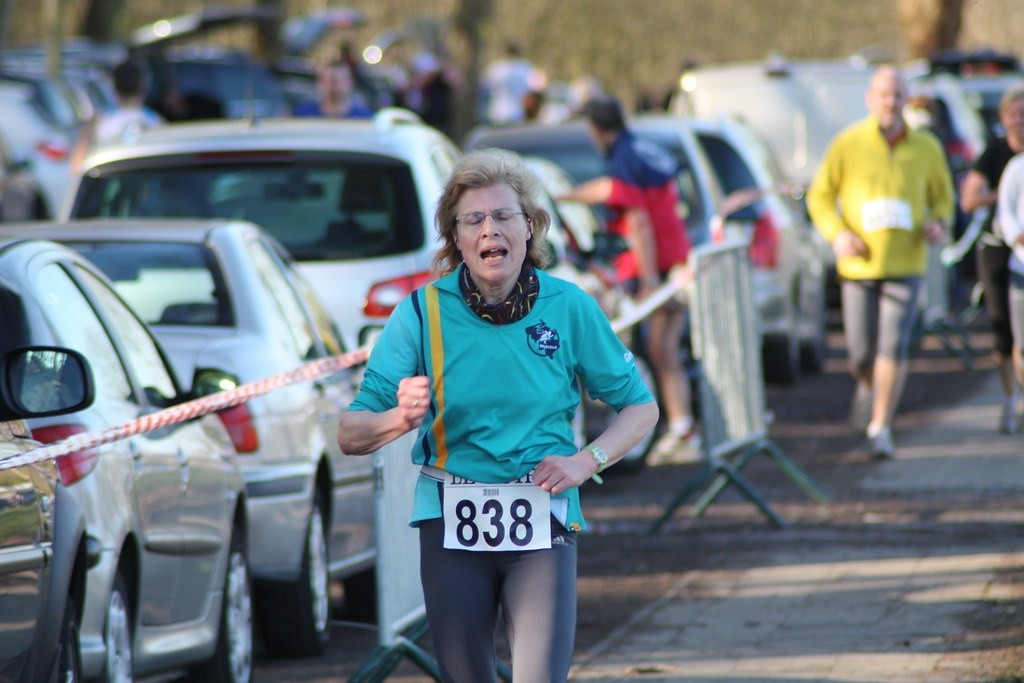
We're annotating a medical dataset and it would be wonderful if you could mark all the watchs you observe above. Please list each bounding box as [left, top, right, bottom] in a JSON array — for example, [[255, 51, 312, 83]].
[[582, 445, 607, 484]]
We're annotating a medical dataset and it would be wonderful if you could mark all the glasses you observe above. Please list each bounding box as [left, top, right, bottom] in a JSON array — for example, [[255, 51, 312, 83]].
[[454, 208, 527, 229]]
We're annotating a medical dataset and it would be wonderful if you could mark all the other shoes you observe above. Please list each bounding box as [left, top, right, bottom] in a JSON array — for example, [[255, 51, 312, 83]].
[[646, 424, 702, 466], [999, 410, 1019, 433], [852, 383, 875, 431], [865, 426, 893, 458]]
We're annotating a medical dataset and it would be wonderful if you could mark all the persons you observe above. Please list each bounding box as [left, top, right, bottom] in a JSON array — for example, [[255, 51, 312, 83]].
[[805, 65, 955, 457], [908, 81, 1024, 436], [71, 36, 696, 174], [338, 149, 660, 683], [550, 96, 701, 463]]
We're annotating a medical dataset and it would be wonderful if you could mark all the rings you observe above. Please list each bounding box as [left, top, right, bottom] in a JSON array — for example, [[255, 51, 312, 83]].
[[413, 399, 419, 408]]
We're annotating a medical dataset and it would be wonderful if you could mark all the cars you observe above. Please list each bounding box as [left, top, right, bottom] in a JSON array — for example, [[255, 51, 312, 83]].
[[0, 234, 258, 683], [0, 220, 388, 658], [0, 36, 1024, 393]]
